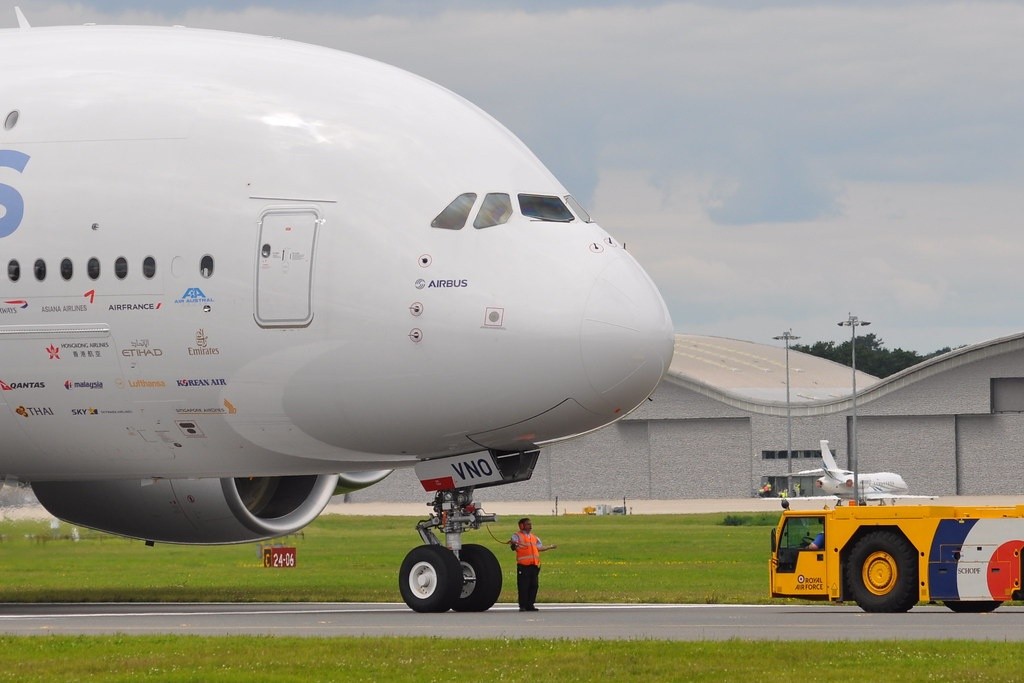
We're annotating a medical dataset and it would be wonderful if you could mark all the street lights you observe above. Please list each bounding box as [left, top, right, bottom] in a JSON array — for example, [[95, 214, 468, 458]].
[[837, 312, 871, 507], [773, 331, 801, 475]]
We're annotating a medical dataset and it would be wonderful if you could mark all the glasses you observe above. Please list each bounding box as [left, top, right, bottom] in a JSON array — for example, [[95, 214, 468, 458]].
[[525, 523, 532, 525]]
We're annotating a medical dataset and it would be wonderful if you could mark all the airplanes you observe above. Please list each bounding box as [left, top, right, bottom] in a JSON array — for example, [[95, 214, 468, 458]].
[[798, 439, 939, 506], [0, 24, 680, 614]]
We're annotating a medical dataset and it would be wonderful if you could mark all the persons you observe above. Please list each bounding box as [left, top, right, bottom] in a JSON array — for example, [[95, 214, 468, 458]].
[[508, 517, 557, 612], [808, 530, 826, 551], [760, 480, 800, 498]]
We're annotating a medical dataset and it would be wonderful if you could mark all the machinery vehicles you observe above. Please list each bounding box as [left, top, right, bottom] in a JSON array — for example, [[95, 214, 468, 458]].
[[769, 504, 1024, 614]]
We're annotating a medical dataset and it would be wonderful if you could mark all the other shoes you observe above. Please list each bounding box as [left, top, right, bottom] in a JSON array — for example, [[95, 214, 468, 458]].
[[519, 606, 539, 612]]
